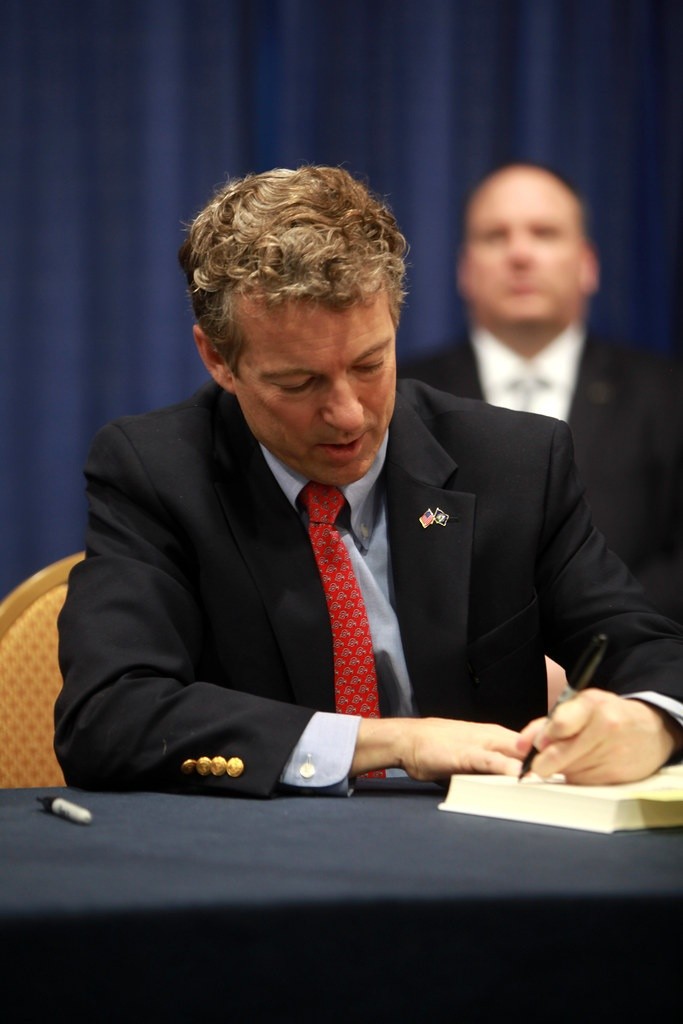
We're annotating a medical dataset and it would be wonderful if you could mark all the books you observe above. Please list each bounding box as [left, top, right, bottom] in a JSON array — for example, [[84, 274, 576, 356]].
[[438, 765, 683, 833]]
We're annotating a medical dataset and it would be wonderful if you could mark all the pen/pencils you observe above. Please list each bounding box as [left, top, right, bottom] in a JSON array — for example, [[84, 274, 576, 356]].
[[516, 634, 610, 784], [35, 795, 93, 825]]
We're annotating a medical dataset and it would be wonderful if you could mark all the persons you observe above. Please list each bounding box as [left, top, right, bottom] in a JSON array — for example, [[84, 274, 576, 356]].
[[391, 161, 682, 482], [53, 168, 683, 801]]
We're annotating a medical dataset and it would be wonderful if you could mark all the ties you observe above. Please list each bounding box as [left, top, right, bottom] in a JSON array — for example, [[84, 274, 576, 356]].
[[300, 480, 386, 779]]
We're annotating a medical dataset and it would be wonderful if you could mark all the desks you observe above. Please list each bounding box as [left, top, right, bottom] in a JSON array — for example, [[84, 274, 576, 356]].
[[0, 790, 683, 1024]]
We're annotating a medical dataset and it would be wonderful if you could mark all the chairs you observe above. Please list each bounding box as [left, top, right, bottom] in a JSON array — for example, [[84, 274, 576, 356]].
[[0, 550, 86, 789]]
